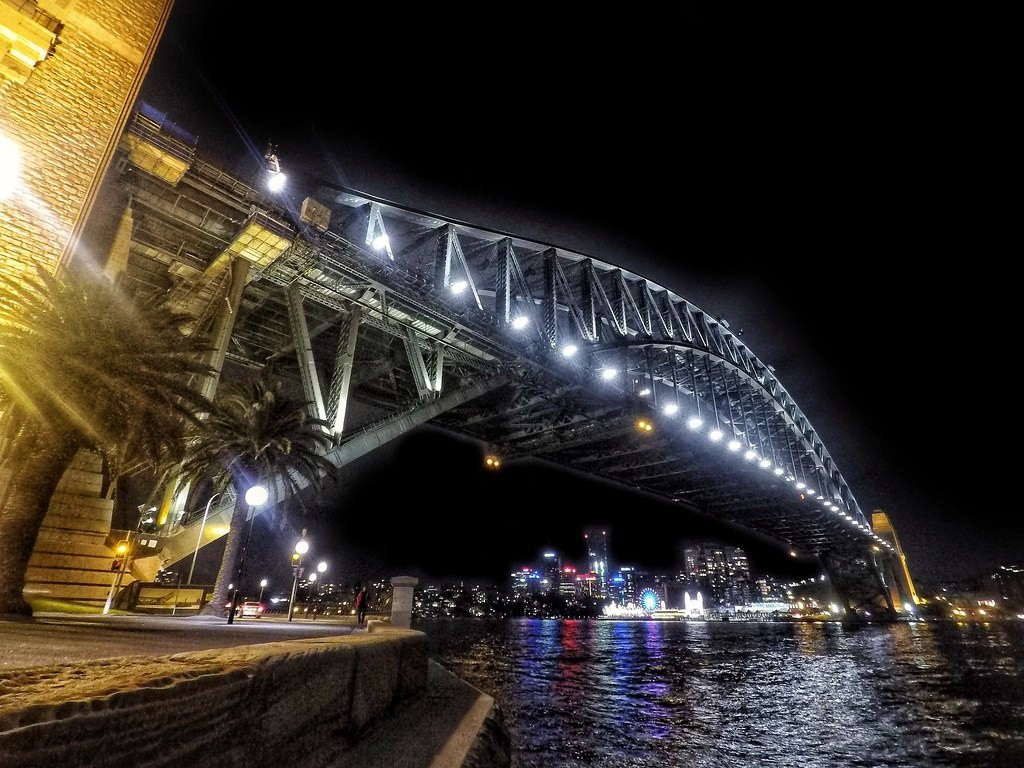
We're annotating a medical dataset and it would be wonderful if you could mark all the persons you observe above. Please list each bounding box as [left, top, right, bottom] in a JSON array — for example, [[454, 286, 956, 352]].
[[357, 586, 372, 629]]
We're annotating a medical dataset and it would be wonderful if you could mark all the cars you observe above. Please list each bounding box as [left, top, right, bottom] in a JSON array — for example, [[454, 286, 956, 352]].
[[238, 601, 265, 619]]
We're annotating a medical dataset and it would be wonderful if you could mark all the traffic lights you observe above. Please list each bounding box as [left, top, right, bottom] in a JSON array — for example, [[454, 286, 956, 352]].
[[113, 539, 130, 559]]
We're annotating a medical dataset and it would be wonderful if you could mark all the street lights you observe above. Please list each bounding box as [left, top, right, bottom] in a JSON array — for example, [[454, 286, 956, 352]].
[[228, 486, 269, 624], [315, 561, 327, 600], [260, 578, 267, 599], [286, 538, 310, 622]]
